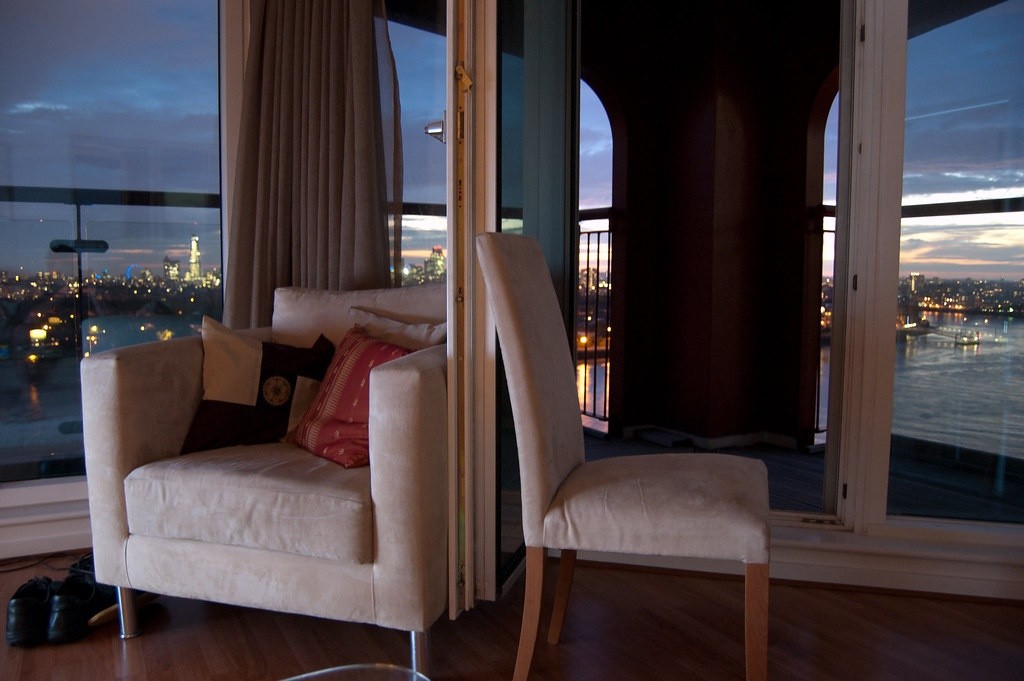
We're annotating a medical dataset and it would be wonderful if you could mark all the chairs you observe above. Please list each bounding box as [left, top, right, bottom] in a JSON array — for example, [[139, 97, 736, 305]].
[[474, 232, 771, 681]]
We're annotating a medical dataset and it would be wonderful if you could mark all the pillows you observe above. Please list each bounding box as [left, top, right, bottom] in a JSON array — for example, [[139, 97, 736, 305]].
[[285, 323, 413, 470], [177, 315, 336, 457], [346, 306, 447, 352]]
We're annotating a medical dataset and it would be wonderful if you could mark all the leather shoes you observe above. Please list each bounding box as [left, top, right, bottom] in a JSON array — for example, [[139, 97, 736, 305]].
[[5, 577, 118, 644]]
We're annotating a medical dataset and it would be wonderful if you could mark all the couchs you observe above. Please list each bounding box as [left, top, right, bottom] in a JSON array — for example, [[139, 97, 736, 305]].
[[79, 282, 451, 681]]
